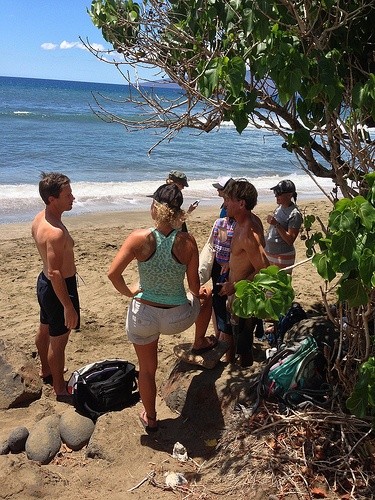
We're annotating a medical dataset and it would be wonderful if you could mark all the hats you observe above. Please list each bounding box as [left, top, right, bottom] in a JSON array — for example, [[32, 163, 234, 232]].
[[269, 180, 296, 192], [212, 177, 233, 188], [147, 184, 183, 211], [169, 169, 189, 187]]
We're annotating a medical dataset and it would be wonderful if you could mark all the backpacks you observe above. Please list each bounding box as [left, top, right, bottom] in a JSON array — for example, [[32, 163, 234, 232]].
[[249, 301, 338, 415]]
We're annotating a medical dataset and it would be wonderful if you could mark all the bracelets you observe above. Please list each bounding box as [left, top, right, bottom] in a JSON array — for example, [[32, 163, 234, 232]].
[[233, 280, 236, 285], [274, 223, 281, 228]]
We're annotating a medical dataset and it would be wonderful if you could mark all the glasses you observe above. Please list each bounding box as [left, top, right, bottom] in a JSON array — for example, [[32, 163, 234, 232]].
[[274, 191, 291, 196]]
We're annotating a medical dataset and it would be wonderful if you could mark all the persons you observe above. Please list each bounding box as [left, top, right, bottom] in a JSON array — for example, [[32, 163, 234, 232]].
[[166, 170, 198, 232], [211, 176, 304, 371], [108, 186, 219, 432], [31, 169, 81, 402]]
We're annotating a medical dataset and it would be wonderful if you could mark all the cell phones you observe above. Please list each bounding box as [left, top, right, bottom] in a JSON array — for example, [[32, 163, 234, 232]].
[[192, 200, 200, 206]]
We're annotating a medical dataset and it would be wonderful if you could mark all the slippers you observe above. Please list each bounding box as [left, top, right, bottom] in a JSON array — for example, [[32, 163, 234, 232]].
[[139, 411, 156, 431], [191, 335, 218, 354]]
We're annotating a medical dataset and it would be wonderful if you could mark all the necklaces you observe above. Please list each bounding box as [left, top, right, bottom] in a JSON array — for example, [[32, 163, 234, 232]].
[[237, 220, 250, 227]]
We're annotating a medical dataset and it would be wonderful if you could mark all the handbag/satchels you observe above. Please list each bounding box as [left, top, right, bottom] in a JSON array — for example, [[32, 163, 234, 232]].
[[67, 358, 140, 412], [198, 218, 219, 285]]
